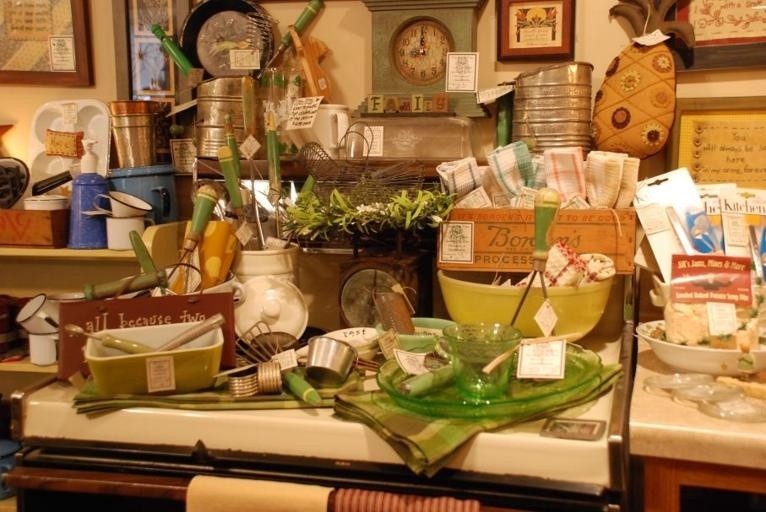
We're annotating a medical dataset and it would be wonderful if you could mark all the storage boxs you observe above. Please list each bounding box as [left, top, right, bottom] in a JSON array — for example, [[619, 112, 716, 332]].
[[433, 199, 637, 277]]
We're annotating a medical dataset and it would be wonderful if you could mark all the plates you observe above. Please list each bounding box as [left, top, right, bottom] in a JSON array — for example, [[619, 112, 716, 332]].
[[306, 327, 382, 361], [636, 322, 762, 376]]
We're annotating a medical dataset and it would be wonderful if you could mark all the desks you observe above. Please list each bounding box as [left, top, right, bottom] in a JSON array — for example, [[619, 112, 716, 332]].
[[628, 315, 765, 512]]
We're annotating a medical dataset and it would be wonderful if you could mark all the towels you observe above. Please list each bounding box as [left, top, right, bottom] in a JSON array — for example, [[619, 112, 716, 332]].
[[185, 476, 484, 512]]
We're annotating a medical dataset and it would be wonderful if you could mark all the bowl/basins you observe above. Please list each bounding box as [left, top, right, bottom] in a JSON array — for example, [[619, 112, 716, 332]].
[[378, 268, 612, 354]]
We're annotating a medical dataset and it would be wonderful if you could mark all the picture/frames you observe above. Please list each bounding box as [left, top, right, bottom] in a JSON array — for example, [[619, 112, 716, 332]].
[[495, 0, 576, 62], [0, 0, 94, 89], [132, 0, 178, 154]]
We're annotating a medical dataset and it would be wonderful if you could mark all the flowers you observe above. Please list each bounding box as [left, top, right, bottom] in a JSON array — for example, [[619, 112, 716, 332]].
[[274, 175, 458, 238]]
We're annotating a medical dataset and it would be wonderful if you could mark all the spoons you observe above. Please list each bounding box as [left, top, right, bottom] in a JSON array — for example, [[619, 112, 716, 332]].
[[64, 322, 155, 355]]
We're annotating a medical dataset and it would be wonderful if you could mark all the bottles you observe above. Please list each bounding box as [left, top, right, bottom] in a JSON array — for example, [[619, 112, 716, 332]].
[[315, 103, 349, 157], [67, 173, 109, 249]]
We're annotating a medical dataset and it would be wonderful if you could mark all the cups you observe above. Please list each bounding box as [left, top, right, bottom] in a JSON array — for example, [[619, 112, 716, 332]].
[[443, 320, 522, 403], [24, 193, 72, 212], [16, 294, 60, 365], [95, 189, 155, 251], [305, 337, 355, 389]]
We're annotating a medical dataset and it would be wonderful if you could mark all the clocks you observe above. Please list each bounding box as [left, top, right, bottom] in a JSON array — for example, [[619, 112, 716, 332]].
[[388, 15, 455, 86]]
